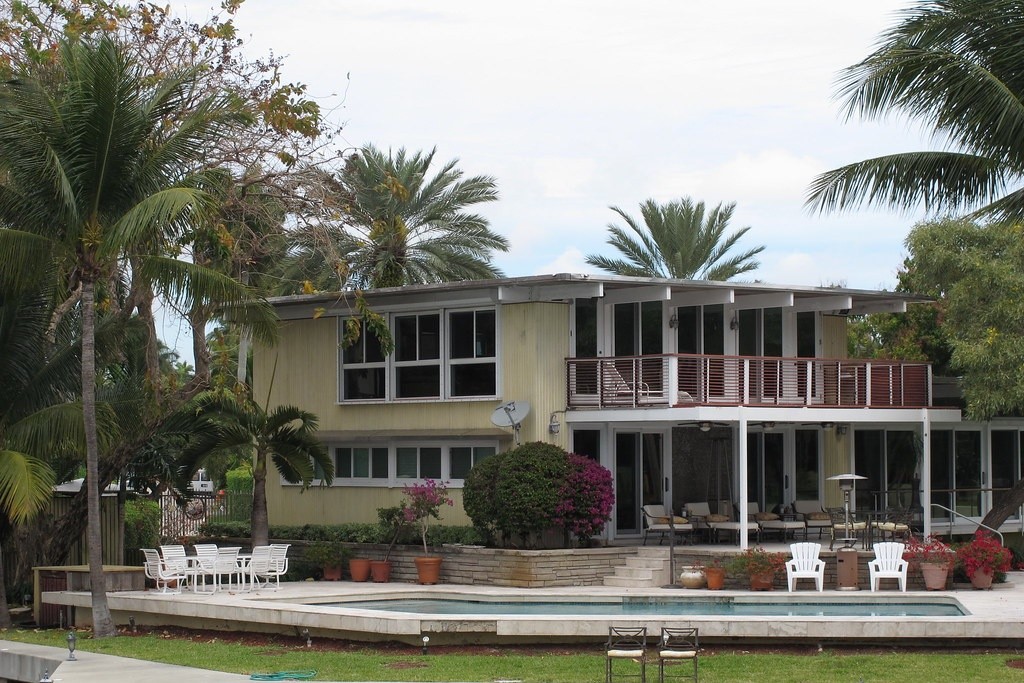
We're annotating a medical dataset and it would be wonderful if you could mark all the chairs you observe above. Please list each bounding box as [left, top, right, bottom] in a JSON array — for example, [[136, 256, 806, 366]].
[[641, 500, 913, 551], [603, 362, 694, 406], [140, 544, 289, 596], [785, 542, 909, 592], [604, 626, 700, 683]]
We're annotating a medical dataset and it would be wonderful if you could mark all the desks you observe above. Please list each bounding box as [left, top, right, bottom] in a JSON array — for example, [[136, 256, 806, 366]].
[[778, 513, 796, 537], [169, 553, 252, 590]]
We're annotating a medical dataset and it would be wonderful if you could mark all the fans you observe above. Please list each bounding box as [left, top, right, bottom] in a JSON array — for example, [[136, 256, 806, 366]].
[[677, 422, 850, 432]]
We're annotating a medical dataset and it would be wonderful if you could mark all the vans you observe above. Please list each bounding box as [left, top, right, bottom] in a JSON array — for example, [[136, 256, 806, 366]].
[[171, 466, 215, 494]]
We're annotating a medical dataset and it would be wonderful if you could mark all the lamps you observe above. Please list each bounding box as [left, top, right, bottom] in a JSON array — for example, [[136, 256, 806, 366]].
[[730, 316, 739, 332], [66, 631, 77, 661], [549, 414, 560, 439], [668, 313, 679, 328], [837, 425, 847, 436]]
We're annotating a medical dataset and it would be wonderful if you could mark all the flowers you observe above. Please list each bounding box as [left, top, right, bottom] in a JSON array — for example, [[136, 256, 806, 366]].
[[402, 441, 615, 554], [905, 528, 1011, 578], [691, 545, 787, 584]]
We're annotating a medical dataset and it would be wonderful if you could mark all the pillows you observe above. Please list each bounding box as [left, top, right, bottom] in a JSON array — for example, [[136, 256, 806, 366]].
[[755, 511, 779, 521], [708, 513, 729, 523], [658, 516, 688, 524], [807, 512, 828, 519]]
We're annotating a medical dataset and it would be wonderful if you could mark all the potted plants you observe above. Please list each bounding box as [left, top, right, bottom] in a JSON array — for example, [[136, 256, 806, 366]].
[[302, 498, 408, 583], [494, 524, 567, 548]]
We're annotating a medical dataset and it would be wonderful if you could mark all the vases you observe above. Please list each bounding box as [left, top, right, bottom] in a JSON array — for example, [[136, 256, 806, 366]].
[[681, 566, 706, 589], [350, 556, 441, 586], [919, 562, 995, 590], [706, 567, 773, 590]]
